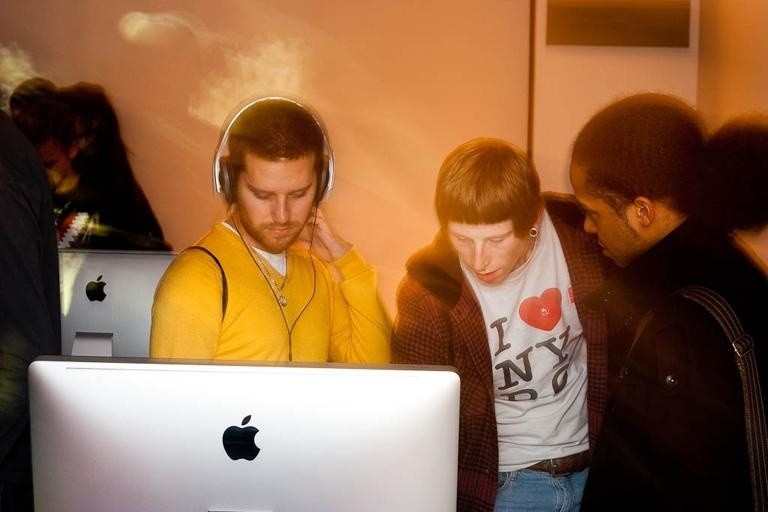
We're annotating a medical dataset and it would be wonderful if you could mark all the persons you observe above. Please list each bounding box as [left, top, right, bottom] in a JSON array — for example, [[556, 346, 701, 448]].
[[569, 94, 768, 511], [11, 81, 168, 250], [708, 114, 768, 271], [146, 98, 395, 367], [0, 107, 61, 510], [396, 137, 627, 511]]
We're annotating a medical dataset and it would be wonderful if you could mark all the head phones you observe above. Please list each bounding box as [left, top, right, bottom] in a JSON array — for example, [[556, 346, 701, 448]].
[[212, 96, 336, 204]]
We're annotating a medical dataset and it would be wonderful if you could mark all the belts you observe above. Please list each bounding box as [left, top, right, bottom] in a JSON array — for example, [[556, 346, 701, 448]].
[[526, 450, 590, 478]]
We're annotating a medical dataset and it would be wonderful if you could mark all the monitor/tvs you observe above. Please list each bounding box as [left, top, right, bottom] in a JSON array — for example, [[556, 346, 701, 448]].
[[27, 354, 463, 512], [58, 248, 179, 357]]
